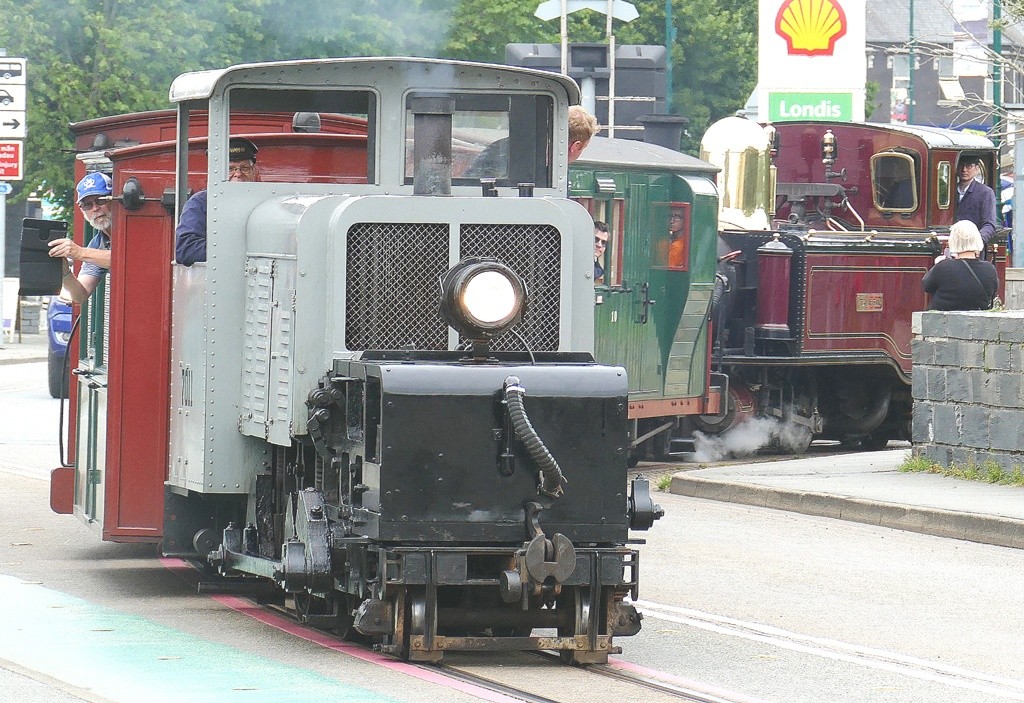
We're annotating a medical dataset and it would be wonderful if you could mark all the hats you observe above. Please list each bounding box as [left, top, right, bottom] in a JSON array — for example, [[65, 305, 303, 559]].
[[76, 172, 113, 203], [205, 138, 258, 160]]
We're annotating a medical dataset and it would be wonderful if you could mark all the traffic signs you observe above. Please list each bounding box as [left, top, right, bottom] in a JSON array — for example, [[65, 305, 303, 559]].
[[0, 57, 28, 139]]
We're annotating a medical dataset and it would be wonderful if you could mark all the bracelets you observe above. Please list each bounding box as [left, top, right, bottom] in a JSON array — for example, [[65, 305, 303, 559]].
[[62, 269, 71, 277]]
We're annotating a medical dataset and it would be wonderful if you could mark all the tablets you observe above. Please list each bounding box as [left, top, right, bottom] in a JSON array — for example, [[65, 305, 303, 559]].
[[18, 217, 69, 297]]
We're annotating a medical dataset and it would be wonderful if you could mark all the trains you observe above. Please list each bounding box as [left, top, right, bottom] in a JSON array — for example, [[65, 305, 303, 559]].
[[48, 55, 1009, 663]]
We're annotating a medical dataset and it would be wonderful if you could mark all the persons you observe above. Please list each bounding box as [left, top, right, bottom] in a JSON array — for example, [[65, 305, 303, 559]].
[[953, 155, 997, 261], [593, 220, 610, 283], [47, 171, 112, 304], [667, 206, 685, 267], [174, 136, 258, 267], [462, 105, 600, 180], [921, 219, 999, 311]]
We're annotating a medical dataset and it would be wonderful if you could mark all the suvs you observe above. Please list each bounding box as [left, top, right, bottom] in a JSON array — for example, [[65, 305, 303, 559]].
[[42, 262, 77, 399]]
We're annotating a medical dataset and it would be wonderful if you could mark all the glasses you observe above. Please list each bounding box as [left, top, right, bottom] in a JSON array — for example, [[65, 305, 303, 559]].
[[959, 163, 975, 170], [594, 236, 608, 247], [229, 163, 255, 175], [80, 198, 108, 211], [666, 214, 683, 223]]
